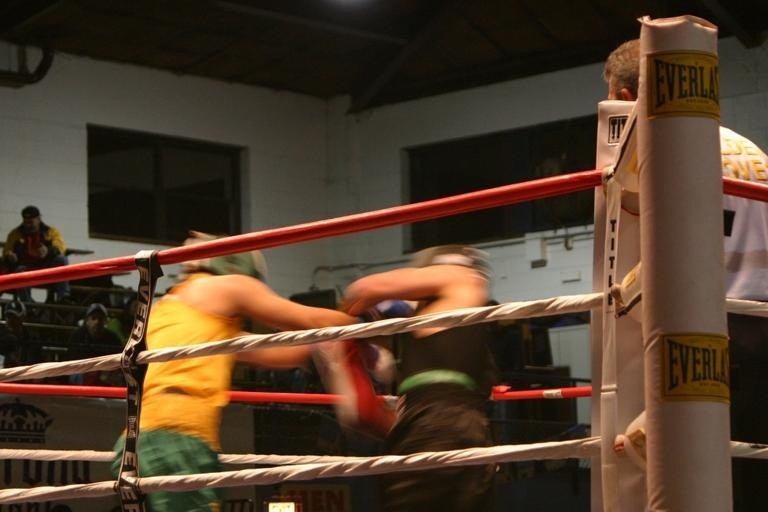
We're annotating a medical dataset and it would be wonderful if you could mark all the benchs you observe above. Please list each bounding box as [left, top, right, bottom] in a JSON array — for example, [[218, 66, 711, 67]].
[[0, 237, 139, 364]]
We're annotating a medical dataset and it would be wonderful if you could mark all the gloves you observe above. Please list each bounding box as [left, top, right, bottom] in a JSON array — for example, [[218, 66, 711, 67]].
[[309, 300, 417, 434]]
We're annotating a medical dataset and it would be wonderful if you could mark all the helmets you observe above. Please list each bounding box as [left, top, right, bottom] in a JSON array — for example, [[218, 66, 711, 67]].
[[417, 243, 492, 275], [184, 234, 267, 283]]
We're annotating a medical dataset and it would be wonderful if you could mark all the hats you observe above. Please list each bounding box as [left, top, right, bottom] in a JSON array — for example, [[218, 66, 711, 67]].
[[86, 302, 107, 317], [4, 300, 27, 317]]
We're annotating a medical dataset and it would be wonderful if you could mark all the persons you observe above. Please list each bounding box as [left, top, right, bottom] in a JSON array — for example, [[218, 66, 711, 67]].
[[112, 231, 360, 512], [2, 205, 548, 395], [599, 36, 767, 510], [342, 247, 492, 511]]
